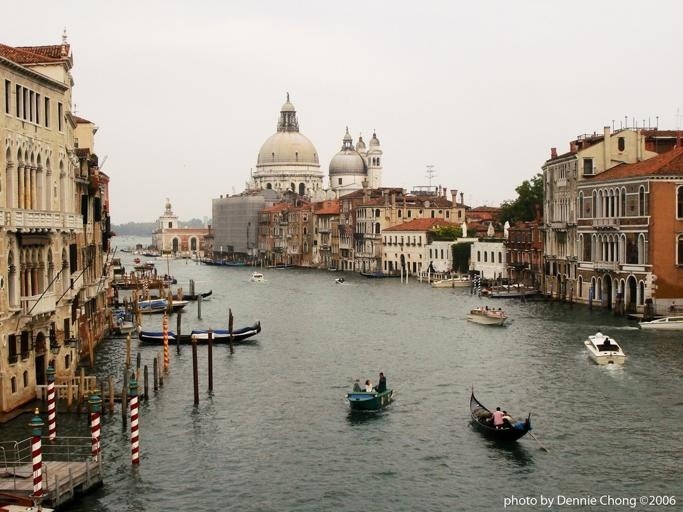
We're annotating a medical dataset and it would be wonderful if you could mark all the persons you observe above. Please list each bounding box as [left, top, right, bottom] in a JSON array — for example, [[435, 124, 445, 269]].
[[378, 372, 386, 393], [492, 407, 504, 429], [353, 378, 361, 392], [365, 380, 372, 392], [604, 338, 610, 351]]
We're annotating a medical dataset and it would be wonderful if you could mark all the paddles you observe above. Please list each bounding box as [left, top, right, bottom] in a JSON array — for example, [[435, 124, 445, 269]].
[[528, 431, 549, 453]]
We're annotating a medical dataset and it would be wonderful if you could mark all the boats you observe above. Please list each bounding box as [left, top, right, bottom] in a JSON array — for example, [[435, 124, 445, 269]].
[[111, 257, 263, 344], [481, 284, 538, 296], [335, 279, 343, 285], [584, 332, 626, 365], [470, 384, 533, 440], [267, 263, 295, 269], [639, 316, 683, 330], [346, 389, 393, 410], [431, 277, 472, 288], [360, 271, 410, 277], [466, 306, 508, 326], [120, 244, 163, 257]]
